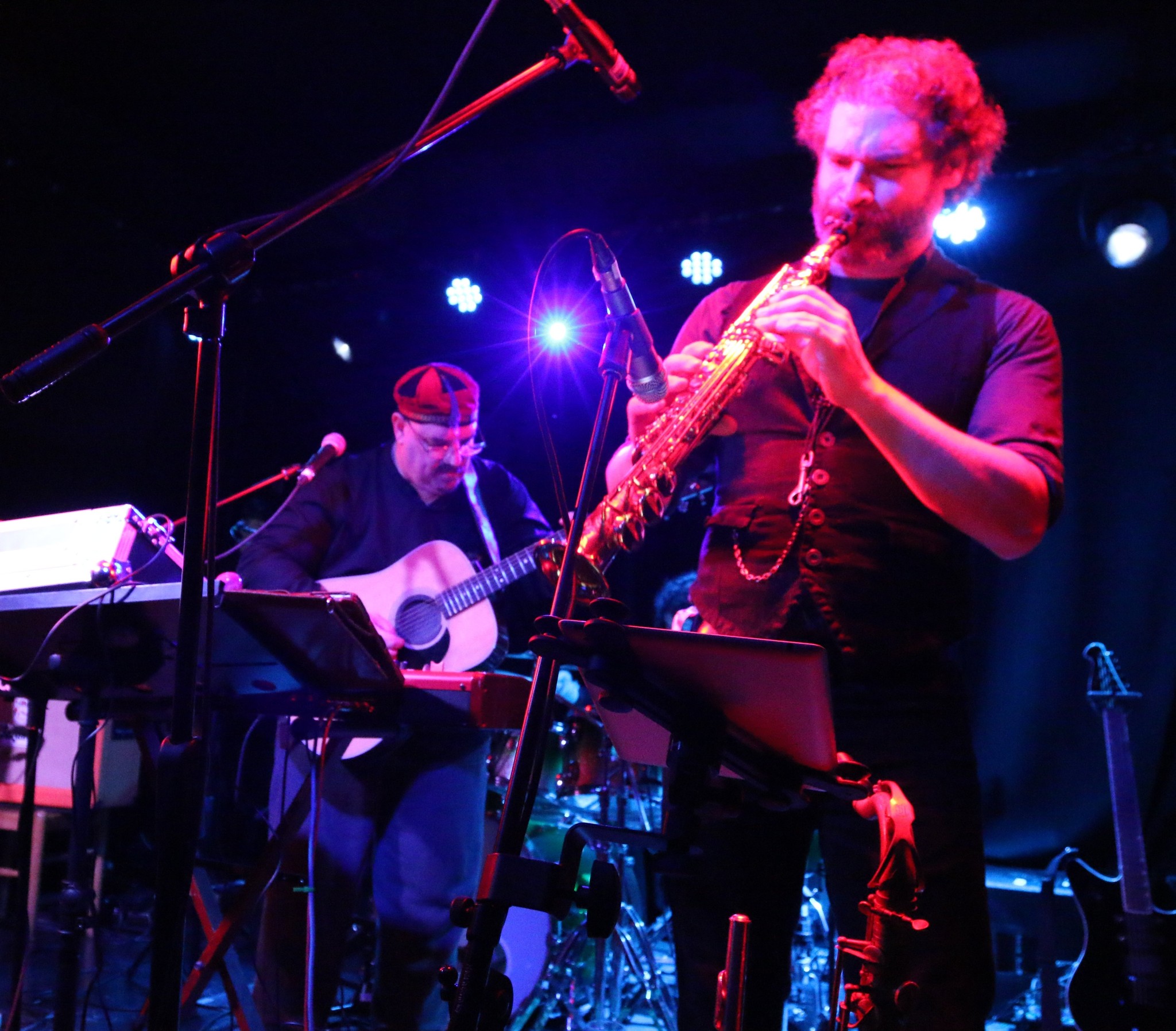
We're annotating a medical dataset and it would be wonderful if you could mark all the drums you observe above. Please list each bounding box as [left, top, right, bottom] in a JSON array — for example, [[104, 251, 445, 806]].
[[415, 831, 570, 1030]]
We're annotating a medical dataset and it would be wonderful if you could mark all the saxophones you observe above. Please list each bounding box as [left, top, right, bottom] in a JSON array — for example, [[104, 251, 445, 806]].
[[530, 215, 866, 604]]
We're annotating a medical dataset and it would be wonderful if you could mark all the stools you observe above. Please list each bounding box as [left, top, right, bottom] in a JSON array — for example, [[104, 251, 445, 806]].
[[0, 805, 113, 970]]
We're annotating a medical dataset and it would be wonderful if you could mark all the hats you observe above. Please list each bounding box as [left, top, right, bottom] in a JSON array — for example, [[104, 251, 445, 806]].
[[393, 363, 479, 427]]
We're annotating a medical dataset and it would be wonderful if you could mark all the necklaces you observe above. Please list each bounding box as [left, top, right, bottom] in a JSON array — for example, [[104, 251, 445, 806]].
[[787, 388, 832, 506]]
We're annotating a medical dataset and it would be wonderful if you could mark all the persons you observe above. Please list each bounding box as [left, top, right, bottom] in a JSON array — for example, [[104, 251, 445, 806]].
[[235, 365, 556, 1031], [606, 37, 1066, 1031]]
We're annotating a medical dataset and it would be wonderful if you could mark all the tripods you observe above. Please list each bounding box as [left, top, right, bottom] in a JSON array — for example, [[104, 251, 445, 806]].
[[508, 750, 679, 1031]]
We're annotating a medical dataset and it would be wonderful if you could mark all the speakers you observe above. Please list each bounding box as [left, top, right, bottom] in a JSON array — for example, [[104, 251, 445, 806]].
[[1, 500, 207, 813]]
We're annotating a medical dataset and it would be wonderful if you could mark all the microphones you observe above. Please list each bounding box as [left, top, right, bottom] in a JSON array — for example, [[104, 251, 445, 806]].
[[586, 230, 671, 405], [297, 429, 360, 478], [545, 0, 640, 104]]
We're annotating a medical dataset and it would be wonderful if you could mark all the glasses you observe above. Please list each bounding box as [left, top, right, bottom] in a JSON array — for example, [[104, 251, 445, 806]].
[[406, 418, 485, 460]]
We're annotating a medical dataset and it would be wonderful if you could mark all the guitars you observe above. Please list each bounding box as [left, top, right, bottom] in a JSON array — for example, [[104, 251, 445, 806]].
[[285, 482, 716, 762], [1038, 631, 1176, 1030]]
